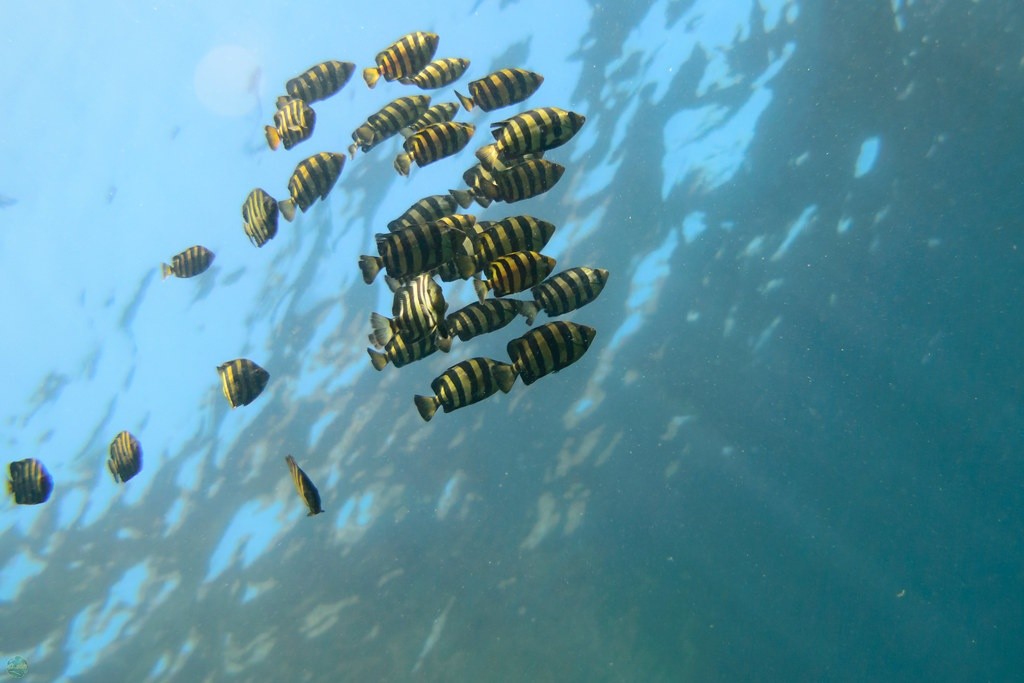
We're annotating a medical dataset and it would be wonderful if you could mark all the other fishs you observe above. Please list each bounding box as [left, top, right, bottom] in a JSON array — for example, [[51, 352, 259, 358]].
[[4, 458, 56, 506], [358, 194, 612, 427], [285, 455, 325, 517], [161, 244, 215, 279], [104, 429, 145, 486], [265, 29, 587, 223], [216, 358, 271, 409], [241, 186, 279, 250]]
